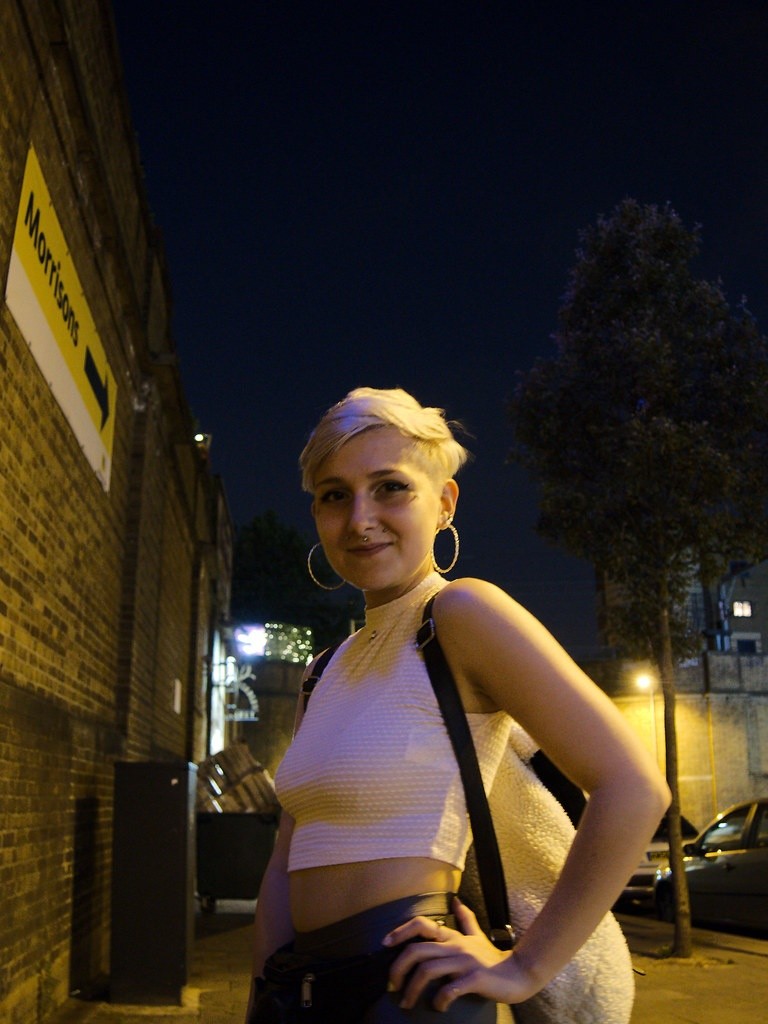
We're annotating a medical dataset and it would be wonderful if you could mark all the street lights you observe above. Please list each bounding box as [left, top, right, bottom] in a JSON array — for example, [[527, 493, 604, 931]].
[[636, 675, 660, 770]]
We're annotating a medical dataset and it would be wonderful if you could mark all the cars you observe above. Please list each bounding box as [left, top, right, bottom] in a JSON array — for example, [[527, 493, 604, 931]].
[[654, 796, 768, 936], [611, 810, 698, 912]]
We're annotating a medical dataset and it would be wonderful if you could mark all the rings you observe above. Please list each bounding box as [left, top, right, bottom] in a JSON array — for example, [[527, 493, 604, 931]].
[[436, 920, 445, 936], [449, 983, 462, 996]]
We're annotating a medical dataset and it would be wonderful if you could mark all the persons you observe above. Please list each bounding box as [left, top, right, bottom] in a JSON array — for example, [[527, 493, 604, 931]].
[[244, 385, 673, 1024]]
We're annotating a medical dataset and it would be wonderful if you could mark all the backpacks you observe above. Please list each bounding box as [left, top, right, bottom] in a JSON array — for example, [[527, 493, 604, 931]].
[[302, 583, 636, 1024]]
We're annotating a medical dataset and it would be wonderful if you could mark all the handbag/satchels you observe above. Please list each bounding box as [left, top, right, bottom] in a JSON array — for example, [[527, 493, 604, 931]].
[[248, 941, 398, 1024]]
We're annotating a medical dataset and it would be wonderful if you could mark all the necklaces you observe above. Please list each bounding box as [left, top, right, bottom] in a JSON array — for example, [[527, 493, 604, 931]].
[[369, 624, 378, 639]]
